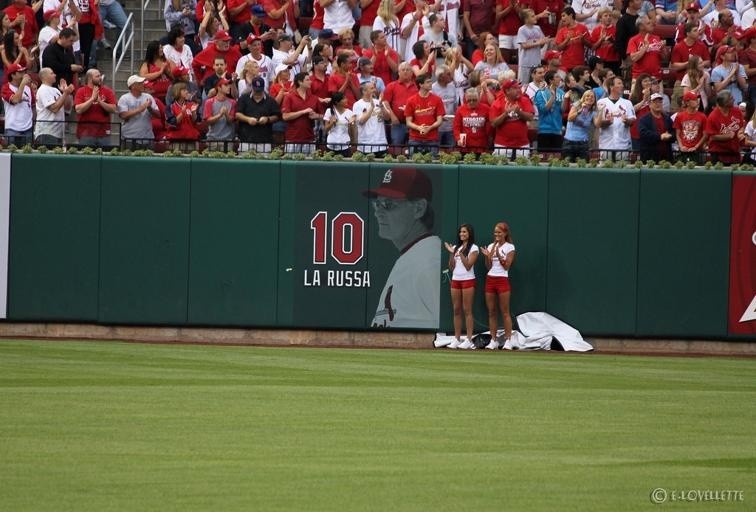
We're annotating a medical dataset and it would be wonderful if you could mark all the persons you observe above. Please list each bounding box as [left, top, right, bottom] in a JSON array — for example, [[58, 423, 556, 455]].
[[445, 223, 479, 350], [0, 0, 756, 167], [480, 221, 516, 351], [360, 167, 442, 333]]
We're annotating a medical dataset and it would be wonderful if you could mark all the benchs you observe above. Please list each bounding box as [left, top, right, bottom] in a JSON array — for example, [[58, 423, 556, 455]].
[[507, 23, 681, 106]]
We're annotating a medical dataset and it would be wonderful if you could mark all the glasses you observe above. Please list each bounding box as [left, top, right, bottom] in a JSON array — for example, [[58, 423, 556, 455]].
[[371, 199, 390, 210]]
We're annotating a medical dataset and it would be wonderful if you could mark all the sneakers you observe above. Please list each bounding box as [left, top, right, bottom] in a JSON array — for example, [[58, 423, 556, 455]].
[[502, 339, 513, 350], [446, 338, 475, 349], [485, 339, 499, 350]]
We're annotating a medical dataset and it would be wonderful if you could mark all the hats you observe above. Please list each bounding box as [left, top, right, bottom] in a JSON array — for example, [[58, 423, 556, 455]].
[[716, 45, 735, 58], [683, 92, 701, 102], [353, 57, 371, 71], [8, 63, 28, 74], [648, 76, 663, 102], [319, 29, 338, 40], [686, 2, 698, 11], [214, 30, 265, 44], [127, 65, 190, 87], [529, 50, 563, 82], [43, 9, 61, 21], [251, 5, 266, 17], [215, 77, 264, 92], [502, 79, 523, 93], [362, 168, 431, 199]]
